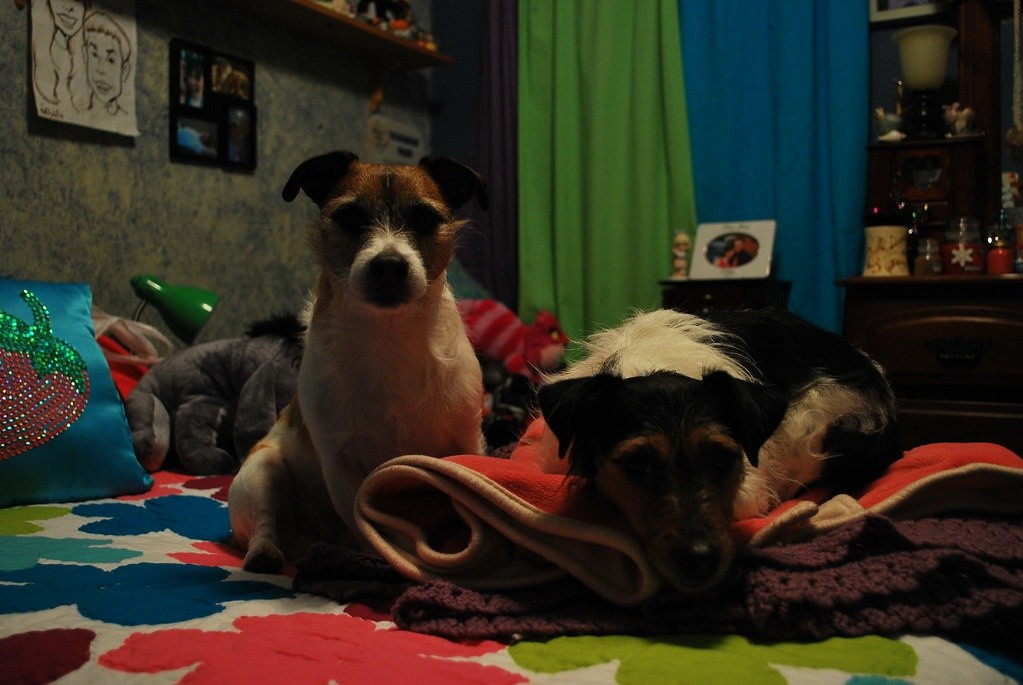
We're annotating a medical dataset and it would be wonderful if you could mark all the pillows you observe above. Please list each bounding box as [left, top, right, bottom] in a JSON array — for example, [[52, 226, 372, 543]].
[[0, 275, 154, 507]]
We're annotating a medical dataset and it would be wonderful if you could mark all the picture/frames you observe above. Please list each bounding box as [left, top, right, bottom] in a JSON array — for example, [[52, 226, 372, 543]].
[[169, 36, 258, 173], [689, 218, 777, 281], [868, 0, 936, 21]]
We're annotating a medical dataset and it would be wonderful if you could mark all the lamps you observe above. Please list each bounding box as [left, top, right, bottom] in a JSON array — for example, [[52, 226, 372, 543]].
[[128, 274, 222, 346]]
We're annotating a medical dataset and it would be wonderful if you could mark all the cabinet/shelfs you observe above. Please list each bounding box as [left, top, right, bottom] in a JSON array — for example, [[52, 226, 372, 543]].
[[834, 0, 1023, 452], [660, 278, 792, 319]]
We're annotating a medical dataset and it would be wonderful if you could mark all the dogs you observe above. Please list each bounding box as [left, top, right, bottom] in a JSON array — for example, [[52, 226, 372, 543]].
[[229, 148, 490, 574], [528, 302, 905, 592]]
